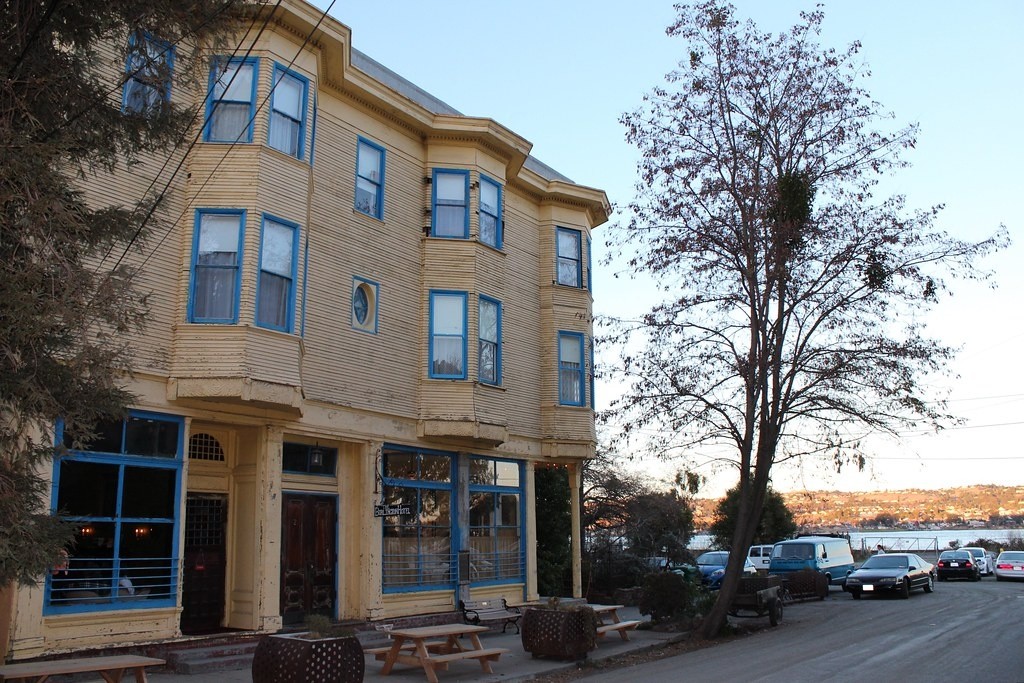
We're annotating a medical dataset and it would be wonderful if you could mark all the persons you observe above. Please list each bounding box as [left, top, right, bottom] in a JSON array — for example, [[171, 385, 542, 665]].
[[1000, 548, 1005, 553], [877, 545, 885, 555], [84, 559, 102, 580], [116, 574, 135, 596]]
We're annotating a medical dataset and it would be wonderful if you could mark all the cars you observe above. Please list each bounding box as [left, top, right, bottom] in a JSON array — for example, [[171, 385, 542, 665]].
[[992, 550, 1024, 582], [956, 546, 994, 576], [673, 550, 758, 593], [843, 552, 938, 600], [935, 549, 982, 581]]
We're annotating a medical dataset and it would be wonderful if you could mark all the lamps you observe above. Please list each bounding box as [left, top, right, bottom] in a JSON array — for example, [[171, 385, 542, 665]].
[[310, 440, 324, 467]]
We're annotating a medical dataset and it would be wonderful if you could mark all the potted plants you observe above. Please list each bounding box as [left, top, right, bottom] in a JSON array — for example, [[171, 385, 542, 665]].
[[251, 615, 366, 683], [737, 571, 778, 595], [520, 597, 598, 663]]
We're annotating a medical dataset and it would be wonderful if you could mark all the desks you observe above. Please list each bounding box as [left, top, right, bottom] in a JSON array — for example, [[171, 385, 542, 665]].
[[379, 624, 495, 683], [0, 655, 165, 683], [589, 604, 632, 641]]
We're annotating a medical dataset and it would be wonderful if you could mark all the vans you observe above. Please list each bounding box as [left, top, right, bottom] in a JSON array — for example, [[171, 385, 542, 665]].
[[762, 534, 856, 591], [747, 544, 782, 570]]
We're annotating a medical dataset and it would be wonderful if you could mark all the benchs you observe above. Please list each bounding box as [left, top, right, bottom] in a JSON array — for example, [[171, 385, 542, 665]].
[[364, 640, 511, 669], [594, 620, 642, 641], [459, 596, 523, 634]]
